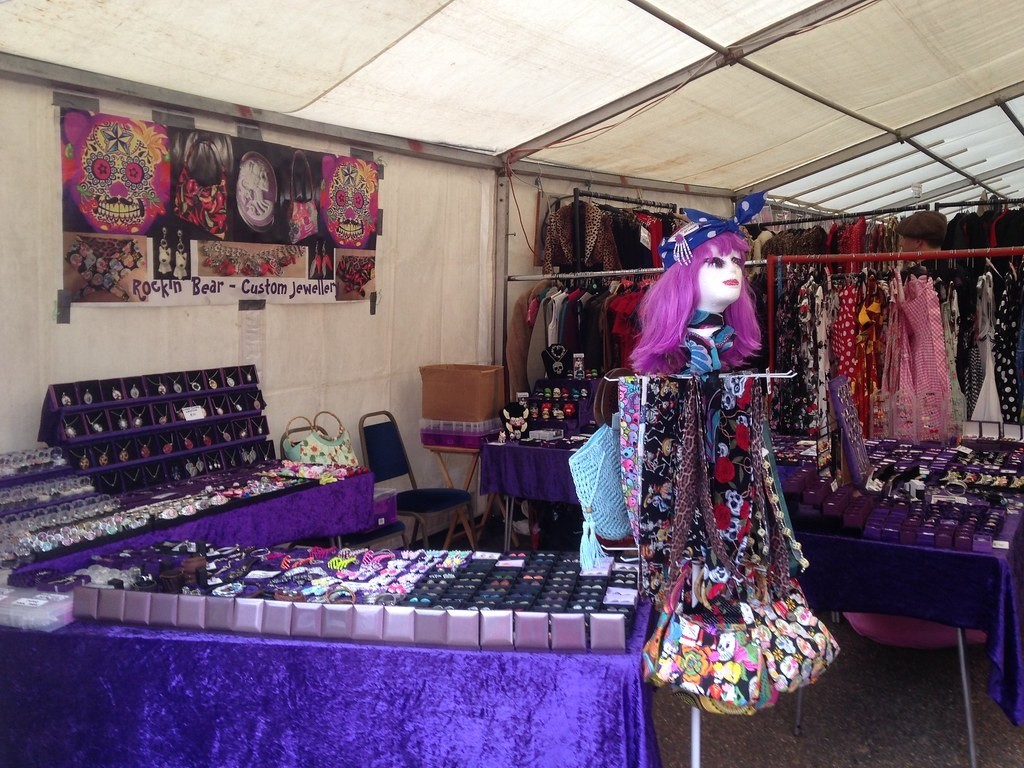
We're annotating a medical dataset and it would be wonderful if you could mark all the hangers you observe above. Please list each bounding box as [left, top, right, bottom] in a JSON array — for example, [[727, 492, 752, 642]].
[[588, 191, 675, 213], [959, 197, 1024, 214], [551, 259, 767, 287], [758, 203, 924, 232], [792, 247, 1017, 271]]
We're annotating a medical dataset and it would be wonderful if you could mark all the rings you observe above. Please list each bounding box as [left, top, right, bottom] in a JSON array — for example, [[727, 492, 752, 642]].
[[409, 569, 484, 610], [789, 468, 1001, 542], [540, 557, 636, 618], [469, 552, 554, 611]]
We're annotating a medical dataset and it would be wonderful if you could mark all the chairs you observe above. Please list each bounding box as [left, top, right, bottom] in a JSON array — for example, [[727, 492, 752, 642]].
[[279, 426, 406, 550], [358, 410, 476, 552]]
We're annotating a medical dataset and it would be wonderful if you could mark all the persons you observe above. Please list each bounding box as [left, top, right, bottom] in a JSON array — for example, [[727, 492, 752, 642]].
[[528, 368, 597, 422], [896, 210, 980, 308], [628, 192, 771, 374]]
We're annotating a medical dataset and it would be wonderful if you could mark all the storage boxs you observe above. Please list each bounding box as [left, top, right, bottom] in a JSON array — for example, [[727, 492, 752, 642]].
[[529, 428, 564, 441], [418, 363, 505, 448]]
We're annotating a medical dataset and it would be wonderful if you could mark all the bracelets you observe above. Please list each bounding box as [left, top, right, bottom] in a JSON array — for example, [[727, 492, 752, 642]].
[[273, 585, 406, 607], [211, 544, 272, 597]]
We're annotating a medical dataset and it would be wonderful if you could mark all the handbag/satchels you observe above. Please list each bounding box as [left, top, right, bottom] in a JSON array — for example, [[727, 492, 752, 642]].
[[171, 138, 230, 241], [738, 588, 841, 694], [282, 411, 359, 468], [282, 150, 319, 244], [567, 368, 633, 571], [642, 560, 780, 716]]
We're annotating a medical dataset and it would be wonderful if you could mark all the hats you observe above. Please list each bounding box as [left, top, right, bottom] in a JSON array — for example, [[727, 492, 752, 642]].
[[894, 211, 947, 245]]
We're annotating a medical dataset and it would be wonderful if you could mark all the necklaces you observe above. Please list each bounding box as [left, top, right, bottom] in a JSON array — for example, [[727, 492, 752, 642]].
[[111, 387, 122, 399], [187, 372, 202, 392], [61, 389, 162, 487], [153, 392, 274, 480], [167, 374, 182, 393], [130, 384, 140, 398], [545, 348, 568, 374], [147, 377, 166, 395], [224, 369, 238, 387], [240, 367, 254, 382], [205, 370, 219, 389]]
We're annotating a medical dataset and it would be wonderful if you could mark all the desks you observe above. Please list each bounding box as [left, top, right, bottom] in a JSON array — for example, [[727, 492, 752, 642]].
[[423, 445, 520, 552], [479, 441, 581, 551], [784, 490, 1024, 768], [13, 472, 375, 573], [0, 598, 663, 768]]
